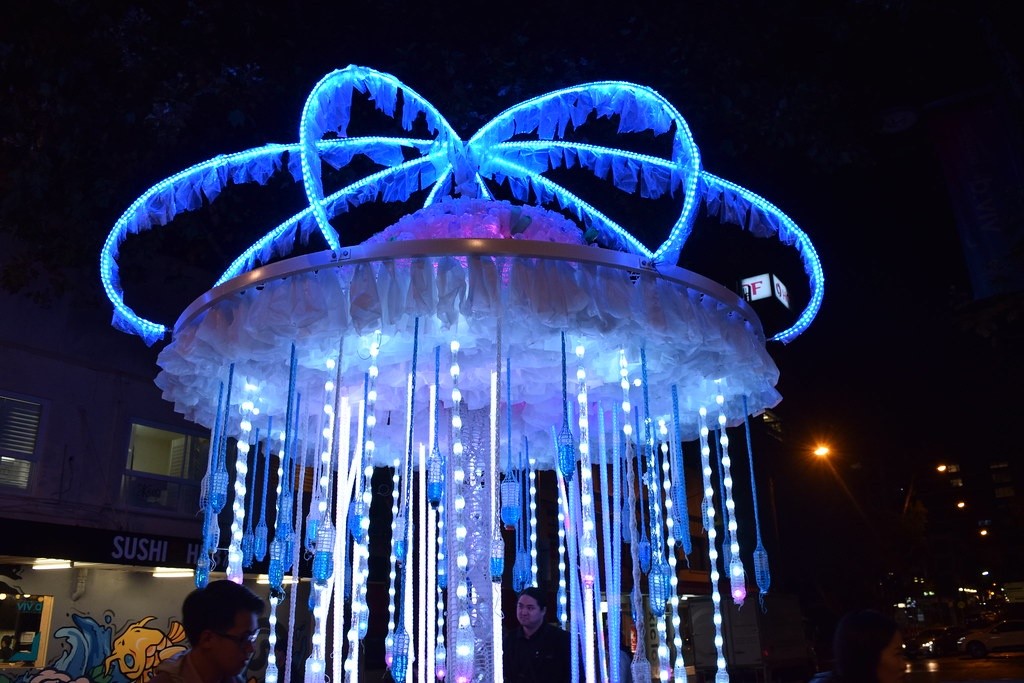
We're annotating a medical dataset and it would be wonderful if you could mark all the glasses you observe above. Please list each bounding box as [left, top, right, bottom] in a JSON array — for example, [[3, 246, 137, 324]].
[[211, 628, 260, 644]]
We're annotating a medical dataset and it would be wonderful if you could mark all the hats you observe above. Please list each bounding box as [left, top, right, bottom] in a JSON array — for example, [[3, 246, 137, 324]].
[[266, 639, 293, 653]]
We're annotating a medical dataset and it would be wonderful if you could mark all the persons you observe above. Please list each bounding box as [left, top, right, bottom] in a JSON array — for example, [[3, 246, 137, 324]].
[[141, 579, 265, 683], [502, 586, 587, 683], [0, 635, 15, 660], [832, 610, 908, 683]]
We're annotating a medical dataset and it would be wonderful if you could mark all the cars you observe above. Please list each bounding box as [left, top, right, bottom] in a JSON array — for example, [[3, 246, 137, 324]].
[[901, 625, 968, 660], [957, 620, 1024, 659]]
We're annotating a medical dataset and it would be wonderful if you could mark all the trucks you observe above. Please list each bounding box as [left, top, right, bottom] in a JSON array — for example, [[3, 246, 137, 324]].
[[687, 592, 818, 683]]
